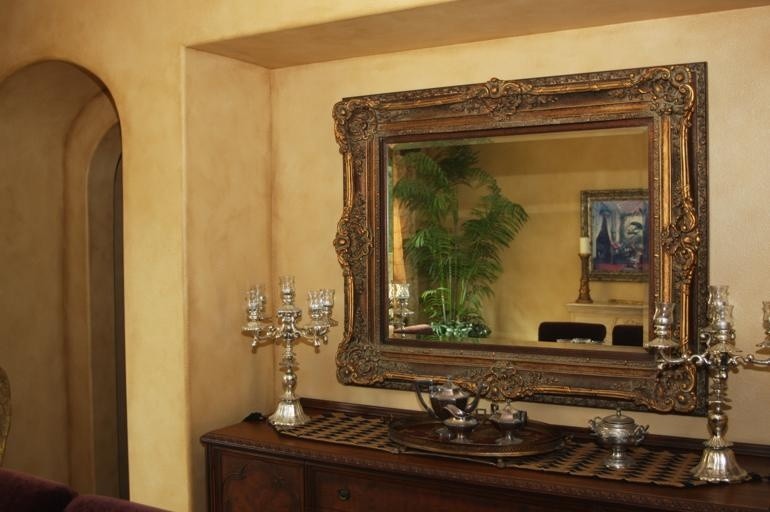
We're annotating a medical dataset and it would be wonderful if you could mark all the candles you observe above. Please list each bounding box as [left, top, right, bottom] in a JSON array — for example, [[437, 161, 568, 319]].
[[580, 237, 592, 253]]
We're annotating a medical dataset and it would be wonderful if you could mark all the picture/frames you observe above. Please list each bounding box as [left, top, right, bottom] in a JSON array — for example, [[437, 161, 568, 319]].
[[579, 187, 650, 283]]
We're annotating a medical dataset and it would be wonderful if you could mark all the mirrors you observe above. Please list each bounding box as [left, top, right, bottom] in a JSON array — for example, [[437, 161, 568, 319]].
[[331, 61, 713, 417]]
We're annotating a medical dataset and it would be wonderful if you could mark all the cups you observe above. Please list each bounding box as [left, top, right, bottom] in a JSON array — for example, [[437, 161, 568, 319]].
[[428, 375, 646, 471]]
